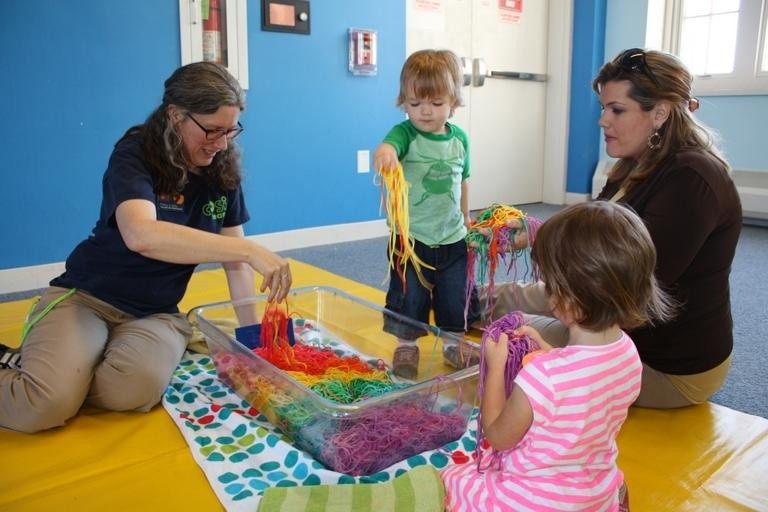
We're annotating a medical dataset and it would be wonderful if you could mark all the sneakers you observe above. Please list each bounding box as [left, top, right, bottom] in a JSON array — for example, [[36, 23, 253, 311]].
[[443, 345, 478, 368], [1, 342, 22, 368], [393, 344, 419, 379]]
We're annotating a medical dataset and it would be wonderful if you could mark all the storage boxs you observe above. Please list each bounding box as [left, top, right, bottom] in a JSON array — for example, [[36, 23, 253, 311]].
[[185, 284, 489, 477]]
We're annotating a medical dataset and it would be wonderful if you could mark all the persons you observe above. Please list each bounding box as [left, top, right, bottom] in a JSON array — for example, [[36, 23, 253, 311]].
[[476, 49, 743, 409], [1, 60, 292, 434], [438, 200, 687, 512], [374, 48, 481, 379]]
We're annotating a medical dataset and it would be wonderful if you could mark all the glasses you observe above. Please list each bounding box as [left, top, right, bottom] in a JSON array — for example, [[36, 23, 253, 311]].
[[612, 49, 660, 88], [187, 112, 243, 140]]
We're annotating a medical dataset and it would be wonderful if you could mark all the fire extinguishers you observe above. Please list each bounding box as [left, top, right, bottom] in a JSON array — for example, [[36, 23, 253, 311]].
[[202, 0, 223, 66]]
